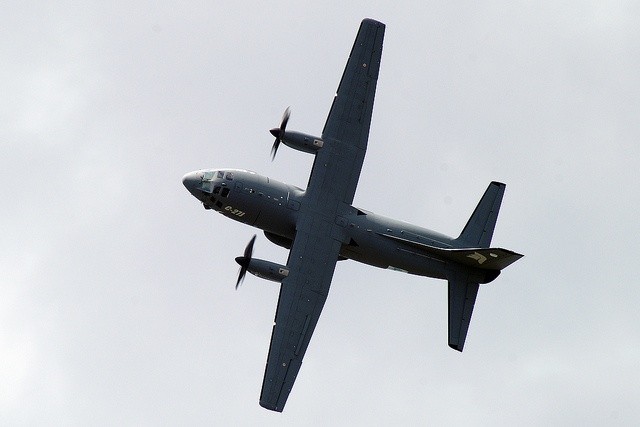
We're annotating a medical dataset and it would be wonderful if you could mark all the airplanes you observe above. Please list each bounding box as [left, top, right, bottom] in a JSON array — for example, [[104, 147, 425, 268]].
[[180, 16, 526, 411]]
